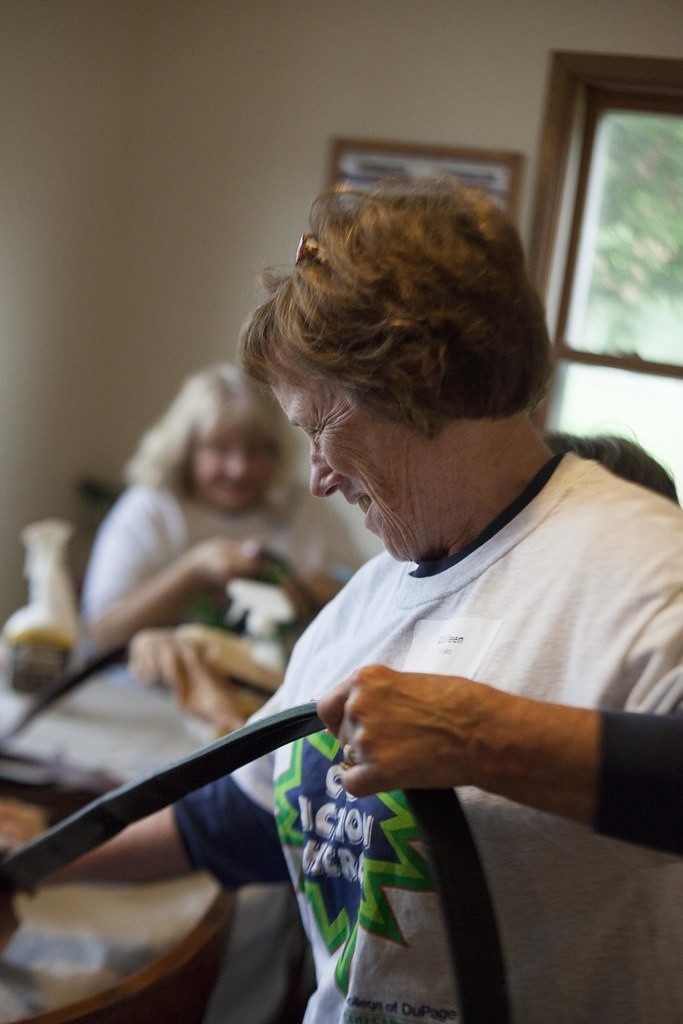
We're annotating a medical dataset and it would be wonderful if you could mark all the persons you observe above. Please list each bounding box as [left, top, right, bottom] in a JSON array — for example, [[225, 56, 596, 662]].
[[2, 173, 683, 1024]]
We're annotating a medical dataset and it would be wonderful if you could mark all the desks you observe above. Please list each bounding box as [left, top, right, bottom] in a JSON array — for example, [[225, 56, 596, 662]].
[[0, 868, 242, 1024]]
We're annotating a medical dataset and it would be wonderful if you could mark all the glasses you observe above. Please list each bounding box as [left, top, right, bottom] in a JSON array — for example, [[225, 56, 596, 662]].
[[294, 234, 332, 271]]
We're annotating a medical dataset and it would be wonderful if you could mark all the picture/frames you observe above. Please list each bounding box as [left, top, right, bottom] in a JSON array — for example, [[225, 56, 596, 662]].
[[323, 138, 529, 238]]
